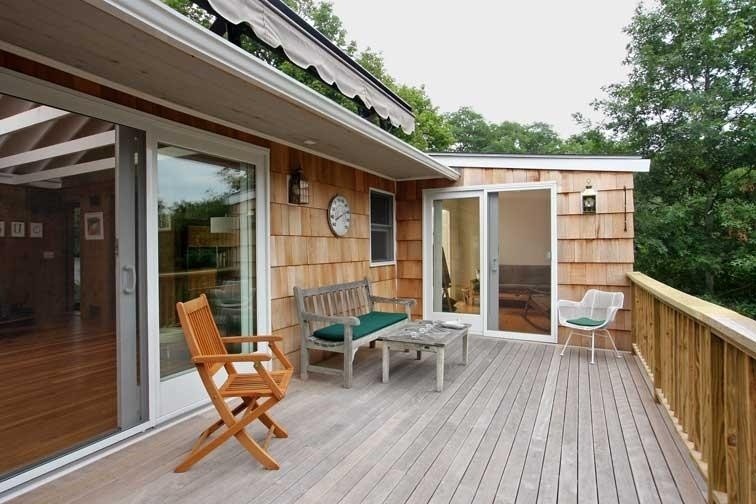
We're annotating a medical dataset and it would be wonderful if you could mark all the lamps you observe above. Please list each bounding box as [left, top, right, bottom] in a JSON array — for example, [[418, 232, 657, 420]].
[[288, 161, 309, 207], [580, 178, 597, 215]]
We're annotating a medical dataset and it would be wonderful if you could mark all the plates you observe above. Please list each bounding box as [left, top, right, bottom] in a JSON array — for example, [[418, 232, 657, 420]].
[[442, 320, 463, 328]]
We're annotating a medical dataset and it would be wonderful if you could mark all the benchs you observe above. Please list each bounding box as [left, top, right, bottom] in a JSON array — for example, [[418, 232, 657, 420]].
[[292, 277, 418, 389]]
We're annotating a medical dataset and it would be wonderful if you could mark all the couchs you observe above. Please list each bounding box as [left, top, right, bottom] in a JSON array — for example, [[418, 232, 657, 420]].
[[469, 266, 551, 306]]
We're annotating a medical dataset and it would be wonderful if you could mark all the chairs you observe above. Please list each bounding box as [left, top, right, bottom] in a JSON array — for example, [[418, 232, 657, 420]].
[[173, 294, 295, 474], [557, 289, 626, 365], [520, 282, 551, 332]]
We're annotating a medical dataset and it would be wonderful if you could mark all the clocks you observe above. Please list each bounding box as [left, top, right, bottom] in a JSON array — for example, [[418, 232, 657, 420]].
[[327, 195, 352, 238]]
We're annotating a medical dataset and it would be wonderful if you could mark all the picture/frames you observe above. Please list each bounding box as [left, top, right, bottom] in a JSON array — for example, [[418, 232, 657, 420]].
[[0, 219, 44, 240], [83, 211, 105, 241]]
